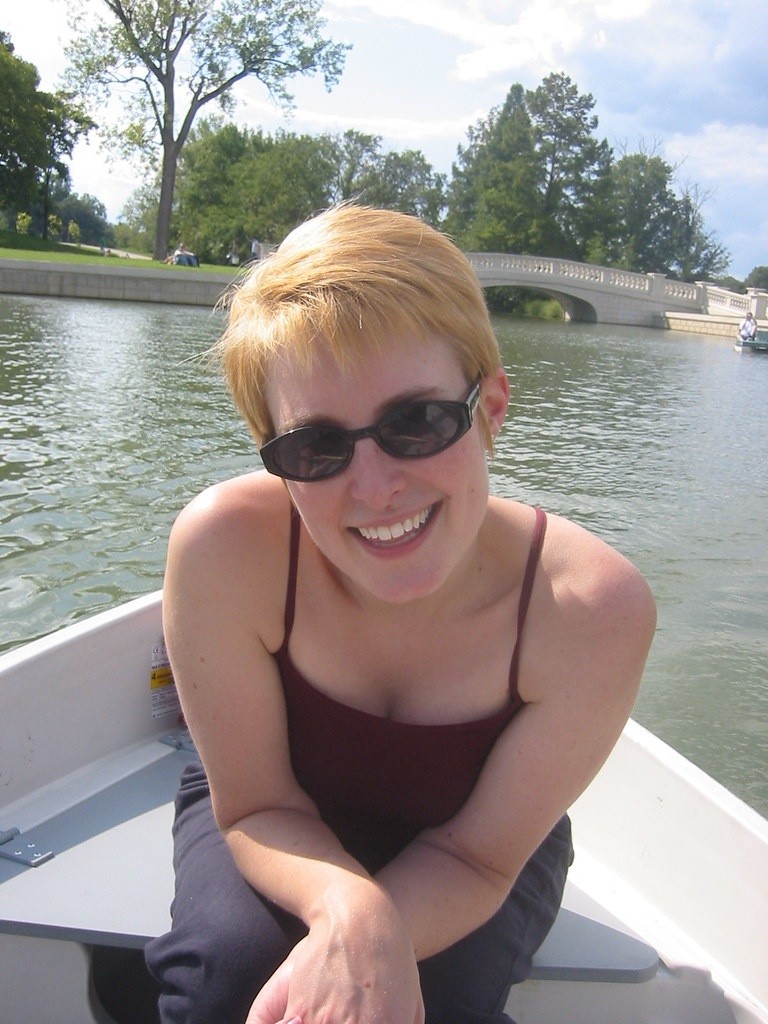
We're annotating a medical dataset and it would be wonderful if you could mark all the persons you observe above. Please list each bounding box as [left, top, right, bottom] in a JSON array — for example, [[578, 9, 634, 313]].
[[143, 206, 657, 1024], [228, 239, 239, 266], [173, 242, 198, 266], [737, 312, 757, 344], [249, 237, 261, 260]]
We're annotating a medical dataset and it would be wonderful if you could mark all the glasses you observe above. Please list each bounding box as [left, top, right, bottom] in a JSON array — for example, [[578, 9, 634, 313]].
[[259, 372, 484, 483]]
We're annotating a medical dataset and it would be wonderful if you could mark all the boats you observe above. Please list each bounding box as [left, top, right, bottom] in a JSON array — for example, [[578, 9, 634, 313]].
[[735, 330, 768, 353]]
